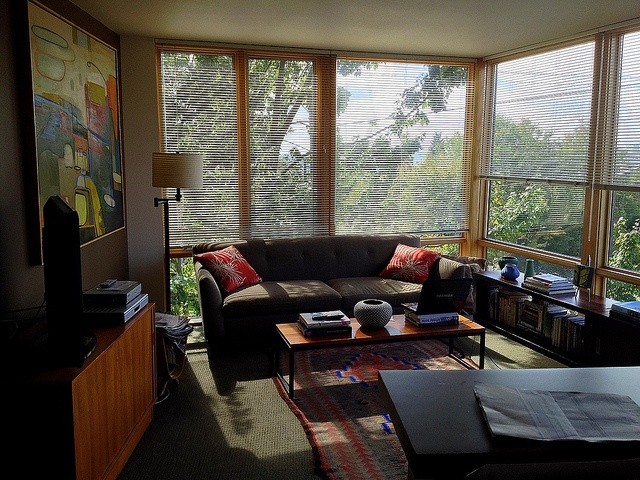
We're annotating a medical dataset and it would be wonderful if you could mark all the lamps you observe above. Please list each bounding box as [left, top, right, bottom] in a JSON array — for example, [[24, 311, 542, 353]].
[[151, 151, 204, 312]]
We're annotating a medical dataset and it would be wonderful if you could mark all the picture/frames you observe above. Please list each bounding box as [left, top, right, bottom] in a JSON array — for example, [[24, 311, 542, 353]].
[[27, 0, 127, 266]]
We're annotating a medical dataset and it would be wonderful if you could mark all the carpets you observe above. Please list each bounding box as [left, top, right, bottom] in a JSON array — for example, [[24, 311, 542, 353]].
[[261, 335, 486, 478]]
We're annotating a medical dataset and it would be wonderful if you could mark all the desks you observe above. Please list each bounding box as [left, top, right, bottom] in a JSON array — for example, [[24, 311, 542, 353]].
[[375, 367, 639, 477]]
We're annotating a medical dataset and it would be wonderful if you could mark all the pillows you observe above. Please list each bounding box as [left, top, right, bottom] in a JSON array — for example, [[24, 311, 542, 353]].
[[380, 243, 441, 285], [192, 245, 262, 296], [192, 235, 471, 364]]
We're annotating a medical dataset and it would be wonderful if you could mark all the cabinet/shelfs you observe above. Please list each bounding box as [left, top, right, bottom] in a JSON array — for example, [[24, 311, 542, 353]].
[[475, 270, 608, 368], [605, 309, 640, 367], [1, 297, 157, 480]]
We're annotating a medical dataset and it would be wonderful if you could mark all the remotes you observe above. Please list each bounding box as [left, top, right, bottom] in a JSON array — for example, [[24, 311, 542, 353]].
[[312, 313, 345, 321], [100, 278, 117, 288]]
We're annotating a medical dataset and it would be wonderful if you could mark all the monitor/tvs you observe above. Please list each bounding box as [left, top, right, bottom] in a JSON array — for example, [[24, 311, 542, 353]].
[[41, 195, 98, 361]]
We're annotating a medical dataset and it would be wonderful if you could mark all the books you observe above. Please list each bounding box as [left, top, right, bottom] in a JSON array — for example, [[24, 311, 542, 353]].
[[610, 310, 640, 326], [521, 273, 576, 295], [488, 286, 532, 328], [542, 301, 592, 356], [611, 301, 640, 318], [300, 309, 351, 329], [296, 319, 352, 337], [517, 300, 543, 335], [404, 309, 459, 328]]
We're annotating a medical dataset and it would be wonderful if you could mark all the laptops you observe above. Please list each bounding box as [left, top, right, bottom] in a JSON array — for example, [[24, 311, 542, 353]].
[[400, 278, 473, 314]]
